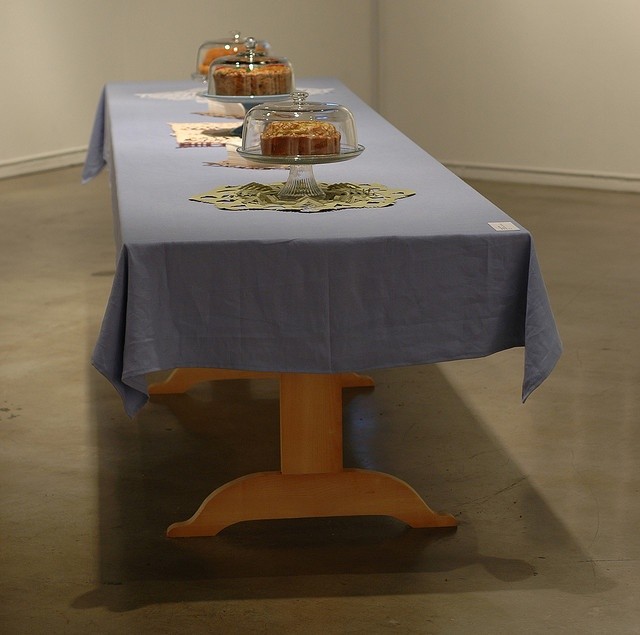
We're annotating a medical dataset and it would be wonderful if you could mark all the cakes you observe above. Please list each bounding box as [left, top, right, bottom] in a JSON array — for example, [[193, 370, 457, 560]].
[[261, 121, 342, 156], [214, 63, 294, 97], [199, 45, 267, 74]]
